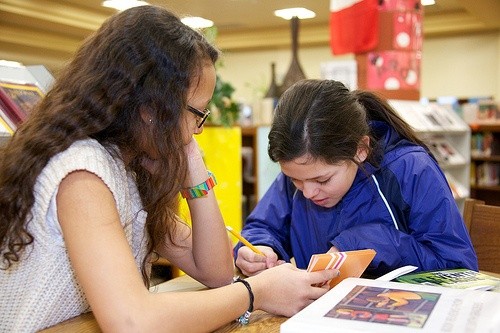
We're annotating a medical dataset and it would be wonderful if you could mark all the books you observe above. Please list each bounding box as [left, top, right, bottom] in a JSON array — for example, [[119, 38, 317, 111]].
[[0, 82, 45, 132], [279, 250, 500, 333], [388, 96, 500, 198]]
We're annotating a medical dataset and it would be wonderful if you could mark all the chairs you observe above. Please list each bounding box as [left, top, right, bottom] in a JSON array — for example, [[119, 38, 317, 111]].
[[462, 197, 500, 276]]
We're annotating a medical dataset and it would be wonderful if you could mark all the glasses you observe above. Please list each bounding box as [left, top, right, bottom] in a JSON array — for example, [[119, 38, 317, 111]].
[[185, 104, 210, 128]]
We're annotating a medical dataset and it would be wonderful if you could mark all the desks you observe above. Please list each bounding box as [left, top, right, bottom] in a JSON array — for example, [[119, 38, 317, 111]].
[[33, 271, 288, 333]]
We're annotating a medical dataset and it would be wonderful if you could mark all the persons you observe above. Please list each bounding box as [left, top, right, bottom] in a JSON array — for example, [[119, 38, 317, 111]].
[[0, 6, 339, 333], [234, 81, 478, 277]]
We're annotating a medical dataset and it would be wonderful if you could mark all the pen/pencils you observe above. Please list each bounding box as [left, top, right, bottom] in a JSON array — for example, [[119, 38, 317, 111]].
[[226, 226, 266, 256]]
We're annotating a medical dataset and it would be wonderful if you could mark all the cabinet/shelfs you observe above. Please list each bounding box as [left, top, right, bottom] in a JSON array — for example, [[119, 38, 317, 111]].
[[418, 119, 500, 212], [152, 122, 283, 279]]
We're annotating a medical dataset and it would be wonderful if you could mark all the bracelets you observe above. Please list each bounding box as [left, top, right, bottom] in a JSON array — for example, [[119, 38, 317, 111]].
[[179, 172, 217, 198], [233, 277, 254, 324]]
